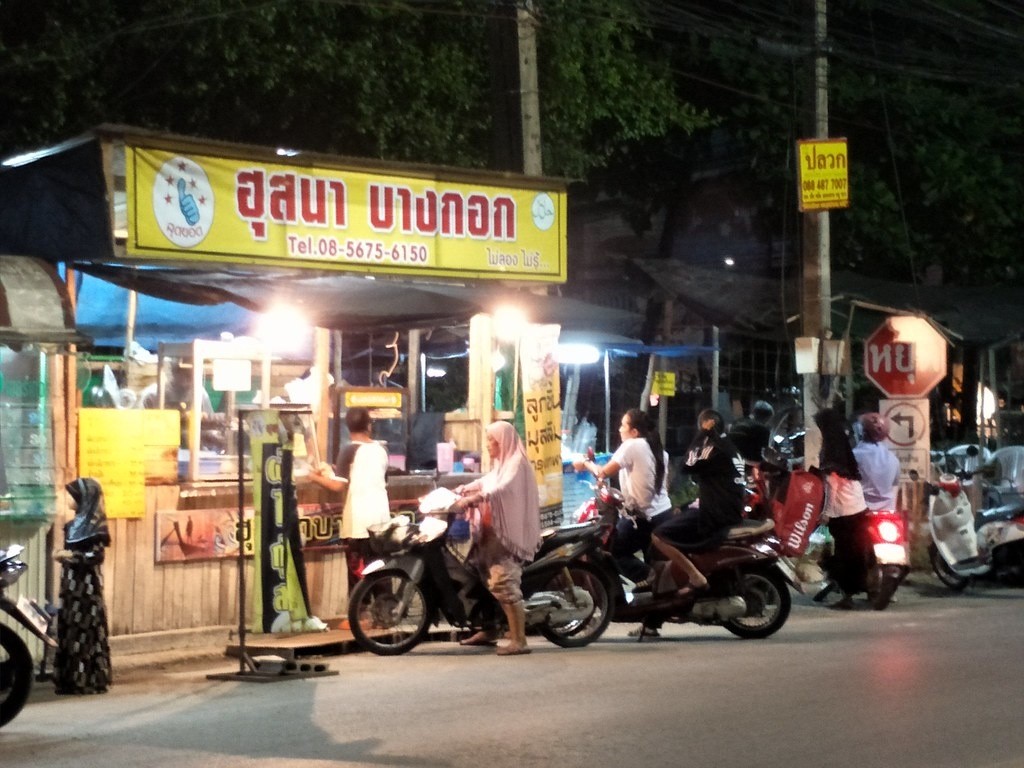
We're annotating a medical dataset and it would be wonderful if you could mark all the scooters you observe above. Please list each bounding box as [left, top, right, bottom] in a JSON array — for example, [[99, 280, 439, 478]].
[[926, 445, 1024, 592], [543, 446, 803, 647]]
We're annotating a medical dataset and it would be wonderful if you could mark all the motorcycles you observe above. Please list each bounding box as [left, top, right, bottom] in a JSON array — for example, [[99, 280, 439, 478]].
[[346, 470, 624, 655], [868, 508, 909, 613], [0, 546, 57, 730]]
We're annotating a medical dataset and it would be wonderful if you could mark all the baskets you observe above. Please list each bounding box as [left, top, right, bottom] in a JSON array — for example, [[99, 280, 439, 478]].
[[366, 520, 415, 553]]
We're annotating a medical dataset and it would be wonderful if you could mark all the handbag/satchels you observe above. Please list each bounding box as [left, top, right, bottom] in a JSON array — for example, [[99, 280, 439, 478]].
[[807, 523, 835, 563]]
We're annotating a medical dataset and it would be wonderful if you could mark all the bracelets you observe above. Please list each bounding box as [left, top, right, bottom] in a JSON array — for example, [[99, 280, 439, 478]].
[[583, 457, 590, 463]]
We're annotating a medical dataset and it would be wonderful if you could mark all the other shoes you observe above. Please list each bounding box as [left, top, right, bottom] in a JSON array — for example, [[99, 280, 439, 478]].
[[628, 626, 660, 637], [630, 580, 653, 593], [337, 609, 372, 629], [374, 614, 392, 630], [827, 599, 855, 610]]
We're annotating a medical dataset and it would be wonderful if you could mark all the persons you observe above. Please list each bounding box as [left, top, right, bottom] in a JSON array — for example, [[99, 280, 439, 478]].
[[650, 409, 746, 599], [574, 408, 673, 637], [727, 401, 901, 609], [418, 421, 544, 655], [52, 478, 112, 695], [305, 407, 393, 631]]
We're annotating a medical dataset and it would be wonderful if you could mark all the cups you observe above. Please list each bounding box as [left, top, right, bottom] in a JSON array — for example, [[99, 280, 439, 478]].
[[437, 443, 454, 472]]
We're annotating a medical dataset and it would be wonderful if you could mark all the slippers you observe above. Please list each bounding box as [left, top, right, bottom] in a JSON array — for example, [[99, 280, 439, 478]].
[[676, 582, 712, 600], [496, 644, 532, 654], [460, 637, 497, 645]]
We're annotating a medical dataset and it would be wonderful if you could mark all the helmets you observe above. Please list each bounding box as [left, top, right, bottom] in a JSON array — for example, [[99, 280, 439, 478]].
[[857, 411, 890, 443]]
